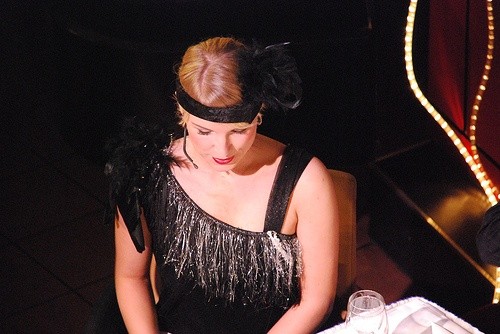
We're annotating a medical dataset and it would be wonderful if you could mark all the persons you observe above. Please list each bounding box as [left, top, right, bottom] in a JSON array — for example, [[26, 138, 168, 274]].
[[104, 37, 341, 334]]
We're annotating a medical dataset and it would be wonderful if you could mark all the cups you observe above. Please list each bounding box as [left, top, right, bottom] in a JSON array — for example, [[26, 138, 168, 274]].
[[345, 290, 389, 334]]
